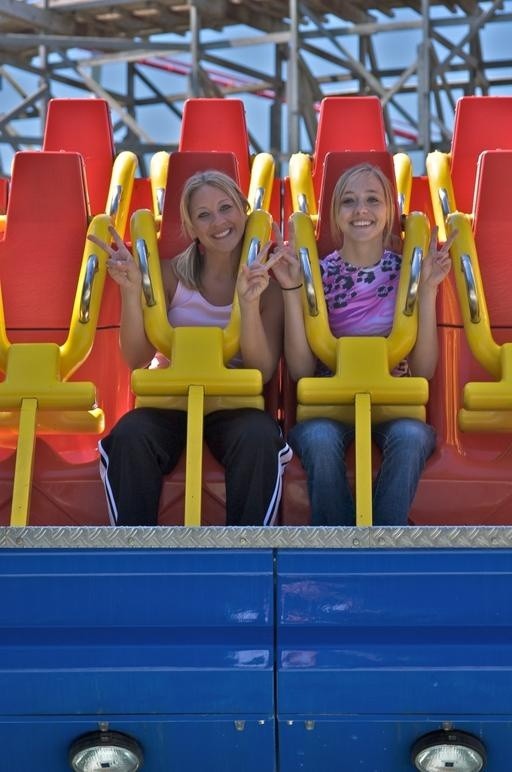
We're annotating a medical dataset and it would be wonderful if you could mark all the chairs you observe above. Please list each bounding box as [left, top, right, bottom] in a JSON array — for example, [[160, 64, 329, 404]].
[[0, 92, 512, 527]]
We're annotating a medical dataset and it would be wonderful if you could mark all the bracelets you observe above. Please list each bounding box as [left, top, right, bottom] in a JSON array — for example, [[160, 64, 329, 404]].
[[278, 282, 305, 292]]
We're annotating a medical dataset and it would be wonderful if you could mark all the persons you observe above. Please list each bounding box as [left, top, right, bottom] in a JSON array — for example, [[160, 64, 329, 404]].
[[267, 160, 460, 525], [87, 169, 284, 526]]
